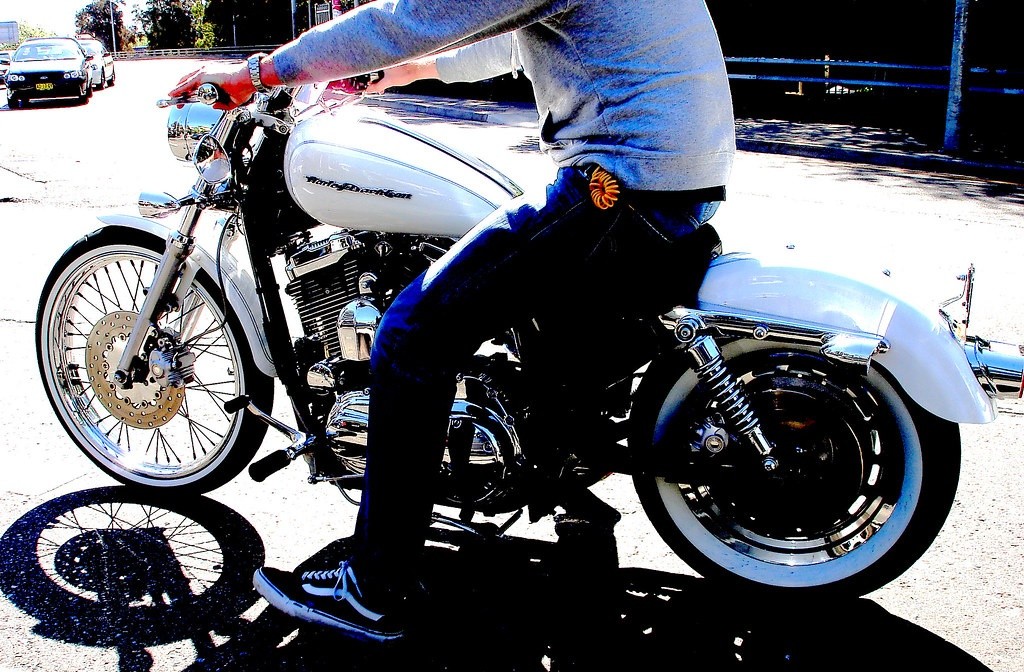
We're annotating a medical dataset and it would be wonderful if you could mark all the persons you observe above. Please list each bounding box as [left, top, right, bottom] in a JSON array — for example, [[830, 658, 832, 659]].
[[167, 0, 737, 638]]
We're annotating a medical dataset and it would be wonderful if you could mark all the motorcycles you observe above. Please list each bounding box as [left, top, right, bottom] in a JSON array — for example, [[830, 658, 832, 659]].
[[33, 70, 1023, 609]]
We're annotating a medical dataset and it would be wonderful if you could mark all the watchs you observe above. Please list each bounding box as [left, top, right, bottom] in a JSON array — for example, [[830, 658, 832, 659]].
[[247, 53, 272, 93]]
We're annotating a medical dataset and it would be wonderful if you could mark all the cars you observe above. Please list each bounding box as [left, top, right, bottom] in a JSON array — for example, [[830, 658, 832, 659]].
[[0, 33, 117, 109]]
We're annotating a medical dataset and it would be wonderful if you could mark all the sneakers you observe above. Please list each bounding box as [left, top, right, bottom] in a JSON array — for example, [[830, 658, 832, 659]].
[[252, 559, 404, 646]]
